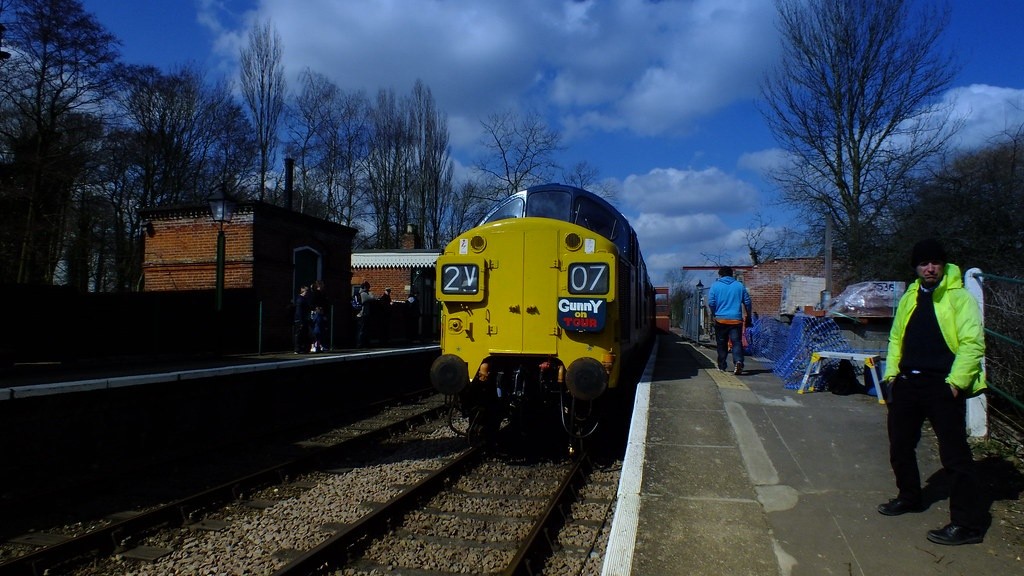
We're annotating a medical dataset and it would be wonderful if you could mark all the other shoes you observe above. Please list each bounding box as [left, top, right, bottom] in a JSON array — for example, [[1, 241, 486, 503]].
[[734, 362, 743, 376], [294, 347, 330, 355]]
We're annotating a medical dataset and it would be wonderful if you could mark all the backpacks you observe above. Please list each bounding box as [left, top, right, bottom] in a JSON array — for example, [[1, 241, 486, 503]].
[[352, 291, 368, 309]]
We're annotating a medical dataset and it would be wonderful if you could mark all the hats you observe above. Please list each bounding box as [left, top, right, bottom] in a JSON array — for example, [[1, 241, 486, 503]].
[[911, 239, 947, 265]]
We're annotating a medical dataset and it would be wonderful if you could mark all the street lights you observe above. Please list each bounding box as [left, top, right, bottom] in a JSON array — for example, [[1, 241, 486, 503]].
[[206, 181, 238, 361]]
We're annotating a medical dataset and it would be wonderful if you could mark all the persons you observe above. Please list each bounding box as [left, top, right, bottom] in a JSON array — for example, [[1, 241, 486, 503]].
[[379, 289, 392, 309], [309, 281, 331, 353], [294, 287, 310, 354], [878, 241, 988, 547], [405, 290, 421, 343], [708, 265, 752, 376], [352, 281, 371, 349]]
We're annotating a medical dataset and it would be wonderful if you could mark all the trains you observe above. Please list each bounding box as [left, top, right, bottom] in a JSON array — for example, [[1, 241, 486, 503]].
[[428, 182, 659, 457]]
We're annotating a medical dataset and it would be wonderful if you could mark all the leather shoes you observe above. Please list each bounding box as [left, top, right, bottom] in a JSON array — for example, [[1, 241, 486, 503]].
[[878, 496, 927, 516], [927, 523, 983, 545]]
